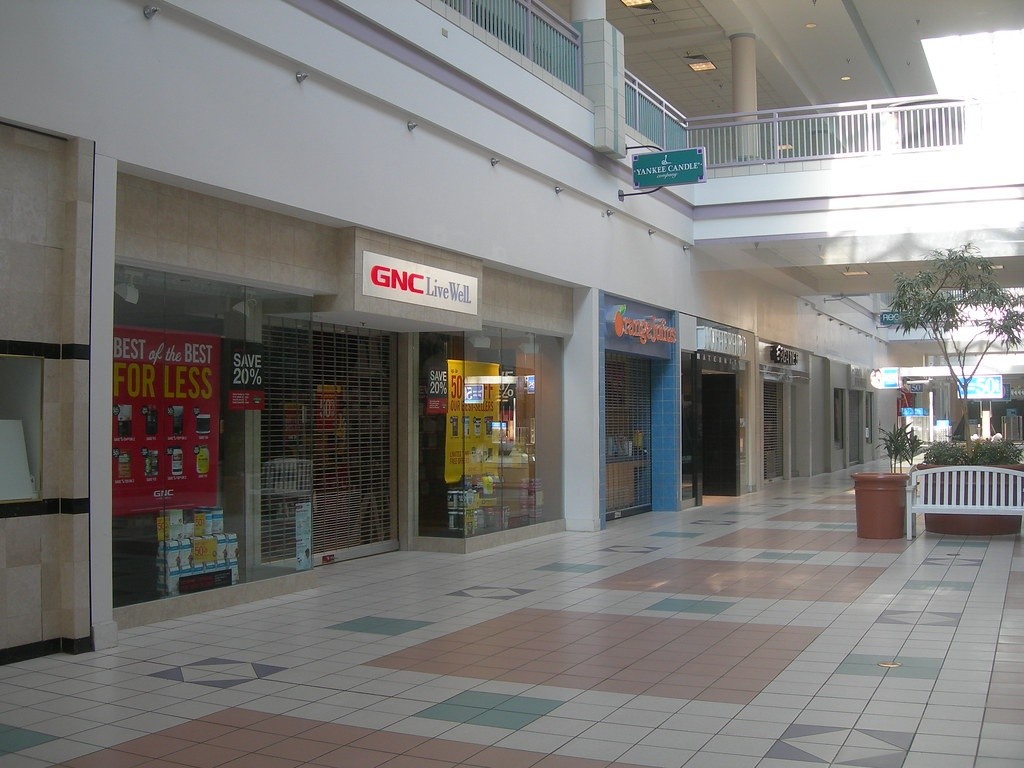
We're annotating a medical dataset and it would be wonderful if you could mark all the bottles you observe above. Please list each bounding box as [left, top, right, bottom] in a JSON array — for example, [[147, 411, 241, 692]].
[[196, 444, 210, 474], [118, 451, 131, 478], [143, 450, 158, 478], [171, 446, 183, 476]]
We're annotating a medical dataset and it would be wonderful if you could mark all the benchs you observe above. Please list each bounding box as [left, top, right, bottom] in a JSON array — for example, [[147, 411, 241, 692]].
[[905, 465, 1024, 541]]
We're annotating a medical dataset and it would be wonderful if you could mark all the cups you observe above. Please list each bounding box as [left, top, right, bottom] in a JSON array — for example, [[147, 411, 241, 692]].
[[146, 405, 157, 434], [173, 406, 183, 435], [196, 413, 210, 434], [117, 405, 132, 437]]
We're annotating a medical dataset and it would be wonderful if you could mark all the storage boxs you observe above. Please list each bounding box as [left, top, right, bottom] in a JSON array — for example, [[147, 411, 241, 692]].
[[446, 489, 509, 530], [155, 508, 239, 596]]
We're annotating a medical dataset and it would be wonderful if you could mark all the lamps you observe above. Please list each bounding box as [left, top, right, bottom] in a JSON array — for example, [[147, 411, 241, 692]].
[[232, 299, 256, 318], [114, 273, 139, 305], [518, 333, 540, 354], [468, 328, 490, 348]]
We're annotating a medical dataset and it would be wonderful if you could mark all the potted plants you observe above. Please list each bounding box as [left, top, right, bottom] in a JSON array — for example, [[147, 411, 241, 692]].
[[851, 423, 923, 540], [884, 241, 1024, 536]]
